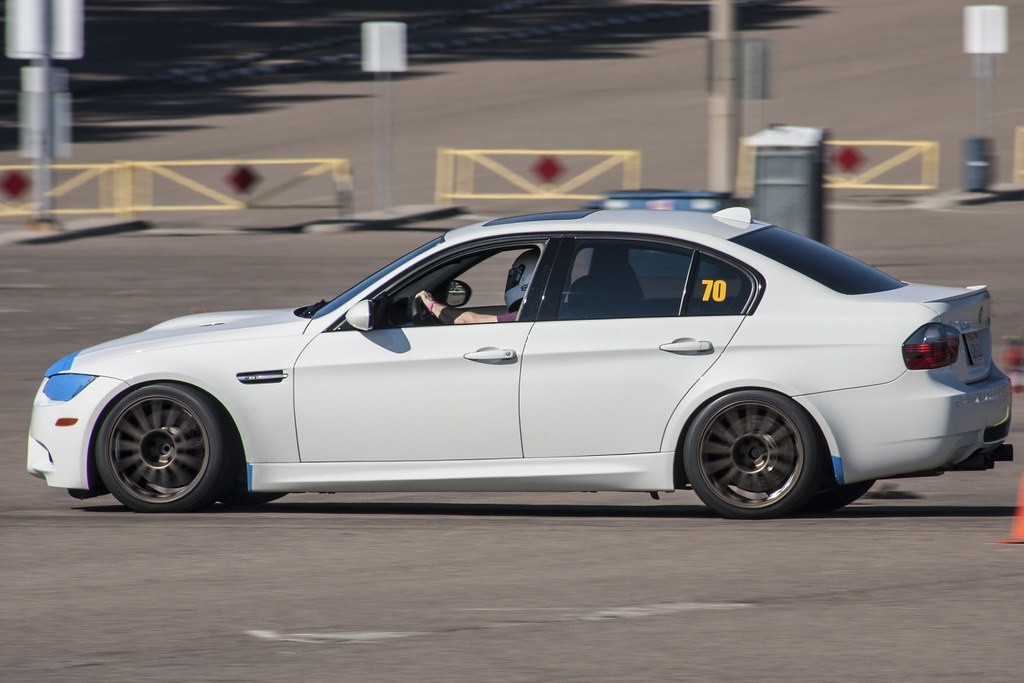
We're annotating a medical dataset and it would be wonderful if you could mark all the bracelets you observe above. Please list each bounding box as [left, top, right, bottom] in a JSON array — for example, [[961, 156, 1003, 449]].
[[428, 299, 438, 312]]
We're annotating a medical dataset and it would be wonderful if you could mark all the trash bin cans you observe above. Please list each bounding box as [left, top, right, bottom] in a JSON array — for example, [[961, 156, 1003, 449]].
[[961, 137, 995, 192], [753, 124, 828, 243]]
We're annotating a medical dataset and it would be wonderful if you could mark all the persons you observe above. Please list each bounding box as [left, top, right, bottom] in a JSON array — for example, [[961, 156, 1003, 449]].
[[415, 248, 542, 325]]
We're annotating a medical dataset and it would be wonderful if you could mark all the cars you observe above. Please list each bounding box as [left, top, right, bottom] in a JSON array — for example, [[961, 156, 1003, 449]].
[[27, 209, 1013, 520]]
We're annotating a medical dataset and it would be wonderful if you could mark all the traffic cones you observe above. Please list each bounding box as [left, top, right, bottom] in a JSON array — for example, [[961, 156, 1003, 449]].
[[993, 472, 1024, 543]]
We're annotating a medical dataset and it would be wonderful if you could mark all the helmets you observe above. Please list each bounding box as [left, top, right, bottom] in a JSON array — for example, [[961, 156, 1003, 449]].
[[504, 250, 540, 312]]
